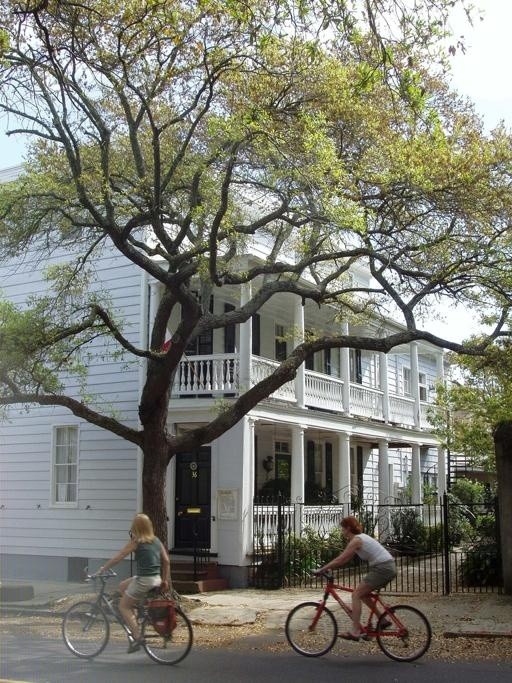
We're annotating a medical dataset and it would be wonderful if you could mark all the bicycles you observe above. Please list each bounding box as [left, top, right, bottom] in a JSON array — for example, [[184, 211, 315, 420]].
[[62, 567, 193, 666], [285, 566, 431, 662]]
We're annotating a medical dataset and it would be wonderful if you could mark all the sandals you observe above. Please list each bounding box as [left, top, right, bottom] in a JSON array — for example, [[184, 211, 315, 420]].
[[126, 636, 147, 654]]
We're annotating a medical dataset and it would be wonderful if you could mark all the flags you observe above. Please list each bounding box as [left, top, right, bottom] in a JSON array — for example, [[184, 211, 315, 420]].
[[161, 327, 173, 353]]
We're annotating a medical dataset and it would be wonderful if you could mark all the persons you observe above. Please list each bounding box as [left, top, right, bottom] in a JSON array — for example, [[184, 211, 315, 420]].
[[312, 516, 398, 643], [91, 512, 171, 654]]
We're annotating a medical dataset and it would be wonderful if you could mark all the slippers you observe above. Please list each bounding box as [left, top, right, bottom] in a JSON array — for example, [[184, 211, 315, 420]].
[[380, 621, 392, 631], [334, 630, 360, 642]]
